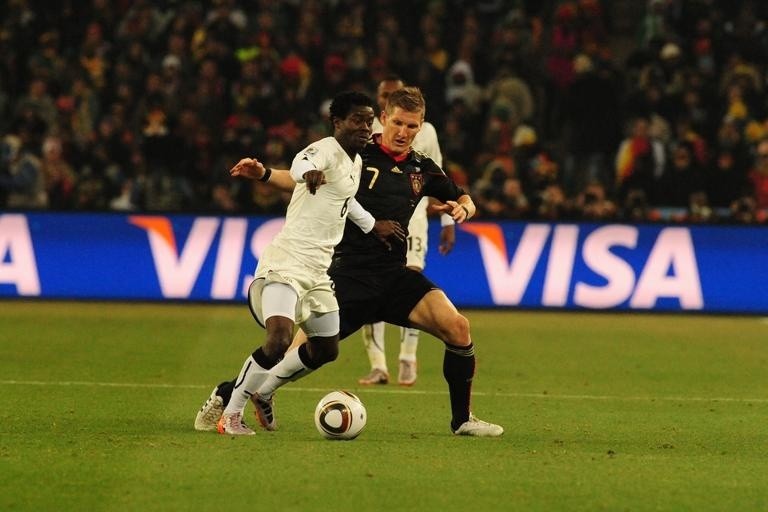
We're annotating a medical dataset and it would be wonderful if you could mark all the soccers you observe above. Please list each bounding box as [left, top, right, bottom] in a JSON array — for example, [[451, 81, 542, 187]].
[[315, 391, 367, 440]]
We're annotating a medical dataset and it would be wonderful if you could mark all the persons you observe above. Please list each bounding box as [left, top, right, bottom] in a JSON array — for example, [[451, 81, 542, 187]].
[[0, 0, 767, 224], [217, 94, 408, 436], [353, 71, 456, 388], [195, 87, 504, 435]]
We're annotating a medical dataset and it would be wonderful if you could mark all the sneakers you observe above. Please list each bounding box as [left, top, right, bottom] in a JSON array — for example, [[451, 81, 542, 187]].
[[398, 359, 416, 386], [194, 382, 275, 435], [451, 412, 504, 437], [359, 369, 388, 385]]
